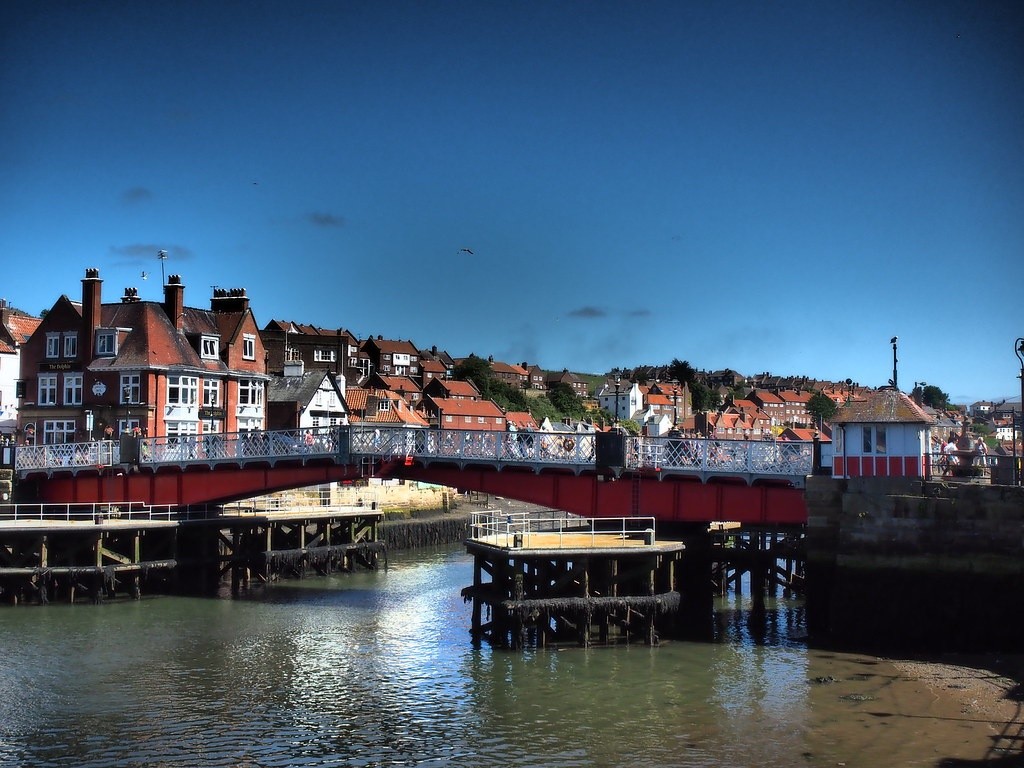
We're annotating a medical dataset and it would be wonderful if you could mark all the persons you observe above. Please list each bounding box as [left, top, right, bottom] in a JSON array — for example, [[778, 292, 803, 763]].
[[944, 437, 960, 467], [188, 437, 195, 459], [374, 426, 381, 452], [305, 430, 313, 446], [685, 431, 717, 465], [504, 421, 535, 457], [972, 437, 987, 465]]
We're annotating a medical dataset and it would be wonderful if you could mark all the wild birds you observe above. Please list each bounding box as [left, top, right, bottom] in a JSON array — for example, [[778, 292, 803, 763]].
[[457, 248, 473, 256], [141, 270, 152, 280]]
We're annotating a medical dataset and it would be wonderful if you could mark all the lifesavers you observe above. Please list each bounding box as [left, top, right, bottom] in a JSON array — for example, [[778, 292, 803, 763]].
[[563, 438, 575, 451]]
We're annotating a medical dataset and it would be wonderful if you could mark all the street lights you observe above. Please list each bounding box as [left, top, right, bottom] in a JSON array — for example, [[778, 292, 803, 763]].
[[607, 370, 623, 435], [122, 384, 133, 437], [209, 392, 220, 433], [667, 377, 682, 436]]
